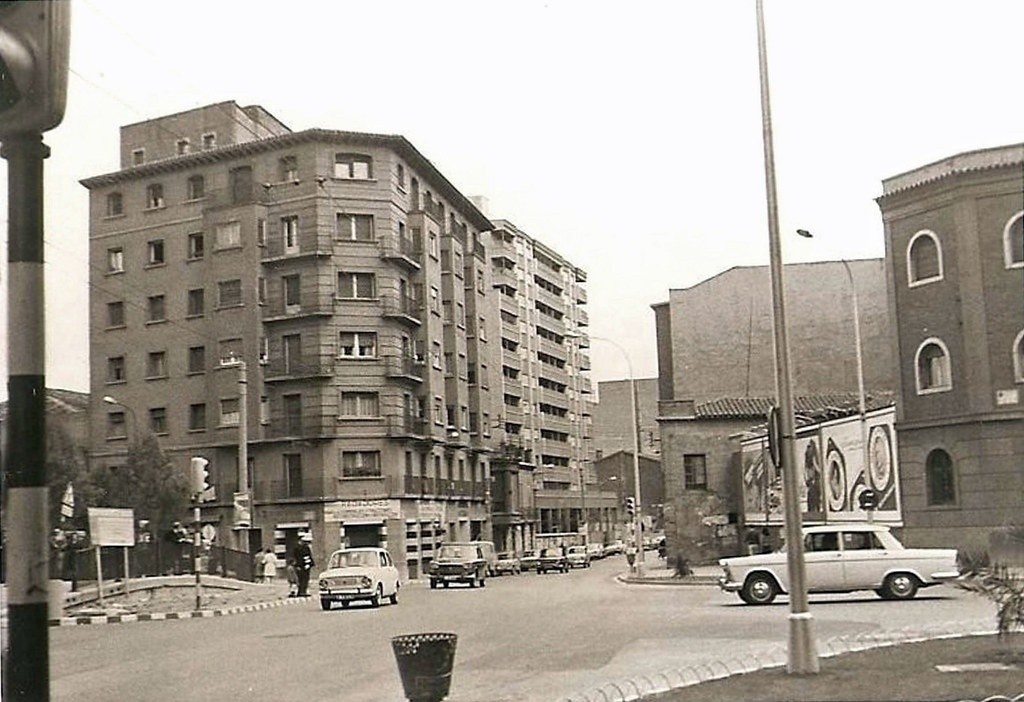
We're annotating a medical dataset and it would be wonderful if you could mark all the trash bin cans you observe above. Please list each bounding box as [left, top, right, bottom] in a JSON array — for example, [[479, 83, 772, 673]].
[[392, 632, 458, 702], [46, 579, 64, 625]]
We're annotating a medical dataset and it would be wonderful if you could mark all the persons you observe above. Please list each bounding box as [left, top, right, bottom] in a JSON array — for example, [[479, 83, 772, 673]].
[[162, 523, 194, 575], [658, 539, 665, 557], [253, 547, 277, 583], [762, 528, 771, 552], [862, 534, 884, 549], [142, 524, 154, 541], [744, 528, 760, 554], [50, 532, 91, 551], [823, 533, 838, 551], [287, 532, 314, 595]]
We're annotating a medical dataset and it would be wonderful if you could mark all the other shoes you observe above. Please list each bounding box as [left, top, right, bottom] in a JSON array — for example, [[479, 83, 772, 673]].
[[298, 594, 311, 597]]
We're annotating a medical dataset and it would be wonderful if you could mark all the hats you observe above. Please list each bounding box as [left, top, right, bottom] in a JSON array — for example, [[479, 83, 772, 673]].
[[301, 533, 313, 541]]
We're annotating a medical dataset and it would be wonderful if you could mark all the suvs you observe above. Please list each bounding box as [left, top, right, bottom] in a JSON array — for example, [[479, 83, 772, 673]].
[[319, 548, 400, 610], [428, 543, 486, 589]]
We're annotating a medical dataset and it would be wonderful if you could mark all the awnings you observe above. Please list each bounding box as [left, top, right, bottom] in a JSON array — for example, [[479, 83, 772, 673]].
[[343, 517, 384, 524]]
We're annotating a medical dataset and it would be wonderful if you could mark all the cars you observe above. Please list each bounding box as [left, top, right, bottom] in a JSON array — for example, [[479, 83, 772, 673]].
[[491, 551, 521, 576], [536, 546, 591, 574], [588, 542, 627, 560], [718, 524, 960, 604]]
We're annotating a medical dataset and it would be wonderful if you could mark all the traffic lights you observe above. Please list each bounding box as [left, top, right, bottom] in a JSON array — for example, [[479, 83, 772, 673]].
[[0, 0, 70, 137], [192, 456, 209, 496]]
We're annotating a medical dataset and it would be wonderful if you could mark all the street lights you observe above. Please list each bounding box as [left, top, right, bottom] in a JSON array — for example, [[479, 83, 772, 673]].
[[797, 229, 875, 521], [101, 395, 139, 447], [561, 331, 647, 575]]
